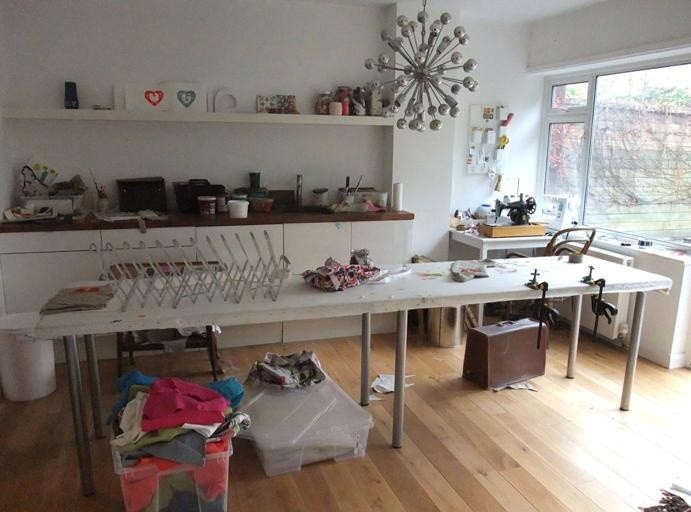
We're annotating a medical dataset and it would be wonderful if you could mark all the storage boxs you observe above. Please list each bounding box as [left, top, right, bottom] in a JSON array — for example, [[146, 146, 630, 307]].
[[107, 419, 234, 512], [231, 367, 372, 477]]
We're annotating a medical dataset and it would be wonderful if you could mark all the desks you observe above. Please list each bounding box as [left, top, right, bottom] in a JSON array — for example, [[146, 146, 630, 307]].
[[34, 252, 671, 494], [450, 227, 557, 331]]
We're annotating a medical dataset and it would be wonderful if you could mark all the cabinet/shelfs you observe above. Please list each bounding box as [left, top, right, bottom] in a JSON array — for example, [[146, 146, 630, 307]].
[[98, 223, 283, 361], [0, 230, 102, 364], [284, 221, 415, 344]]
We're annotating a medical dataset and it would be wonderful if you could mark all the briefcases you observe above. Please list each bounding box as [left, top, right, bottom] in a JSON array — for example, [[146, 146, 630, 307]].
[[460, 316, 550, 392]]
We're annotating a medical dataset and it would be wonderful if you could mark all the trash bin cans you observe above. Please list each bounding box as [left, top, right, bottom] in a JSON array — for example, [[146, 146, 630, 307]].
[[0, 311, 56, 402]]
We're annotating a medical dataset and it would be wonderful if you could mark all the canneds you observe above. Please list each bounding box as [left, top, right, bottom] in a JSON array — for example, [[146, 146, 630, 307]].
[[337, 86, 367, 115]]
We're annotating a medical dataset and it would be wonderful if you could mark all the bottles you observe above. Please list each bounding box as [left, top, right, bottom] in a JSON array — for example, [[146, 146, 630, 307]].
[[312, 188, 329, 209], [296, 174, 303, 210], [315, 81, 383, 115], [197, 194, 274, 219]]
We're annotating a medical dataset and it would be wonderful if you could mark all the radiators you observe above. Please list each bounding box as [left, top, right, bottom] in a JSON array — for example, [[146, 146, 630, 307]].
[[553, 235, 634, 352]]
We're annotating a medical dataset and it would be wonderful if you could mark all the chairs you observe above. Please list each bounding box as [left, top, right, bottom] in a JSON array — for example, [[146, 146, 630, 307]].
[[500, 225, 597, 343], [95, 260, 227, 385]]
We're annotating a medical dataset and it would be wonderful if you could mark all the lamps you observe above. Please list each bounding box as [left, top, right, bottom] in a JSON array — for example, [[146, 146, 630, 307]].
[[366, 0, 480, 133]]
